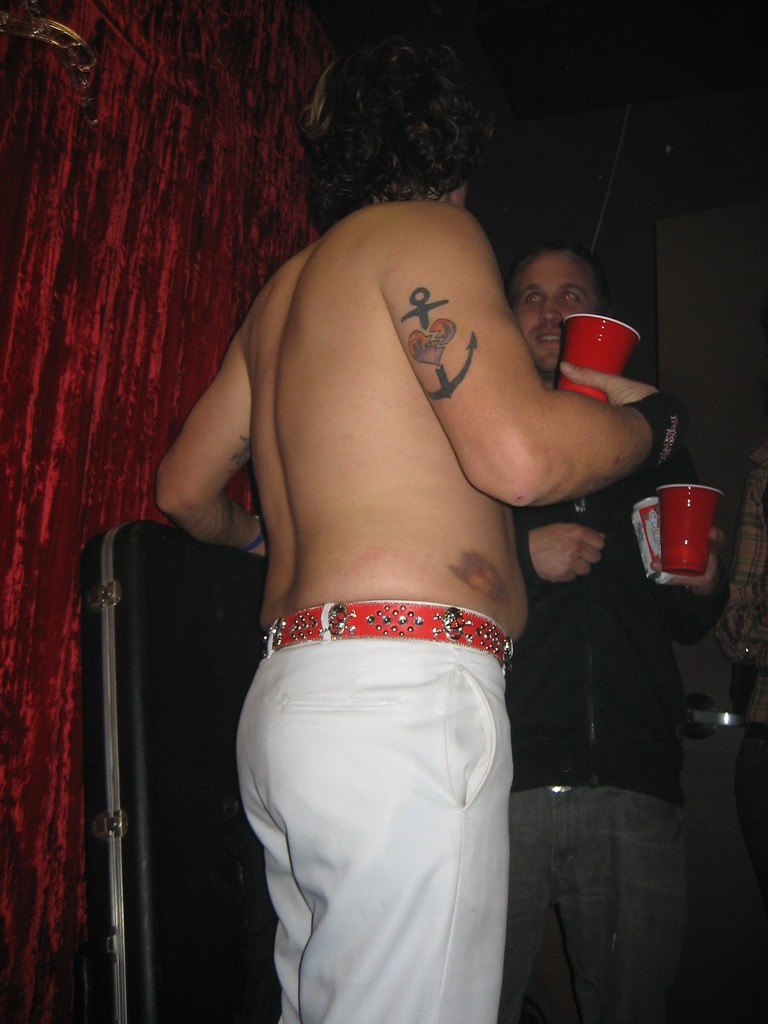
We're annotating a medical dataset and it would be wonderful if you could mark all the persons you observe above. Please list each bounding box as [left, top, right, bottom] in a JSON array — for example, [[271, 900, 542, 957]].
[[712, 434, 768, 903], [500, 240, 732, 1023], [155, 37, 692, 1023]]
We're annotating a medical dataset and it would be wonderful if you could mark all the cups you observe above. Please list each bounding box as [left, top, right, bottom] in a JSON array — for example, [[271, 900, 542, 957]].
[[657, 484, 724, 576], [557, 313, 640, 404]]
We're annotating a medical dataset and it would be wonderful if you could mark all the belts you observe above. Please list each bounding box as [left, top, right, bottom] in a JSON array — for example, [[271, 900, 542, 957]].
[[262, 602, 512, 670]]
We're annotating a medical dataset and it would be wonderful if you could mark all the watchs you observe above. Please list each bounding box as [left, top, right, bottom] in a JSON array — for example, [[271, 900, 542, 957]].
[[239, 511, 266, 554]]
